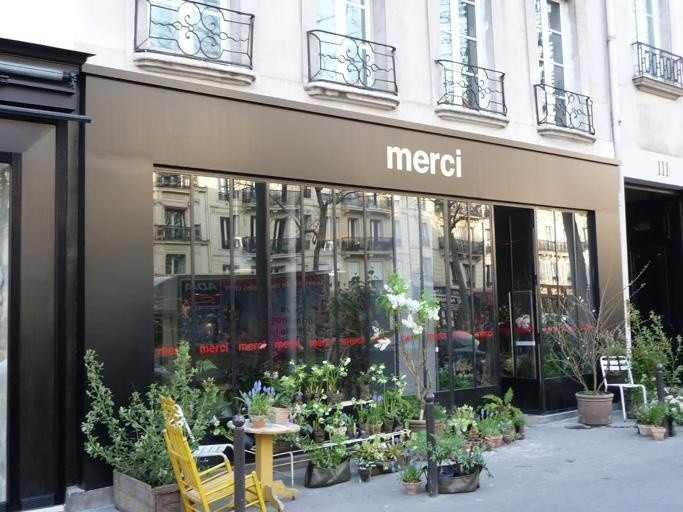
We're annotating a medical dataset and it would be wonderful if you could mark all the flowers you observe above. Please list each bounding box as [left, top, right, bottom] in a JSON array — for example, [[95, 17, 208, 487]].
[[264, 355, 421, 474], [372, 270, 441, 419], [239, 380, 277, 414]]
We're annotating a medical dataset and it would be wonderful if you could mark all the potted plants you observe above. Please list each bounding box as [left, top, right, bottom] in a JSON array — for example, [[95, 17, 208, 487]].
[[635, 402, 668, 440], [81, 340, 222, 512], [399, 387, 525, 493]]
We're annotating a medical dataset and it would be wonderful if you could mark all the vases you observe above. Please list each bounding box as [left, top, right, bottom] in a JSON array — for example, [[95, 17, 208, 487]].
[[250, 415, 266, 427]]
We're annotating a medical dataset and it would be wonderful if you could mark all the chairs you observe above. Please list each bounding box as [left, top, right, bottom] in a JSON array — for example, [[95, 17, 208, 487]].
[[600, 355, 647, 420], [173, 404, 234, 496], [161, 396, 268, 512]]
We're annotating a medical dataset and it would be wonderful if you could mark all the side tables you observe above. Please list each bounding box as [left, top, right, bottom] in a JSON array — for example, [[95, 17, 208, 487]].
[[228, 420, 301, 512]]
